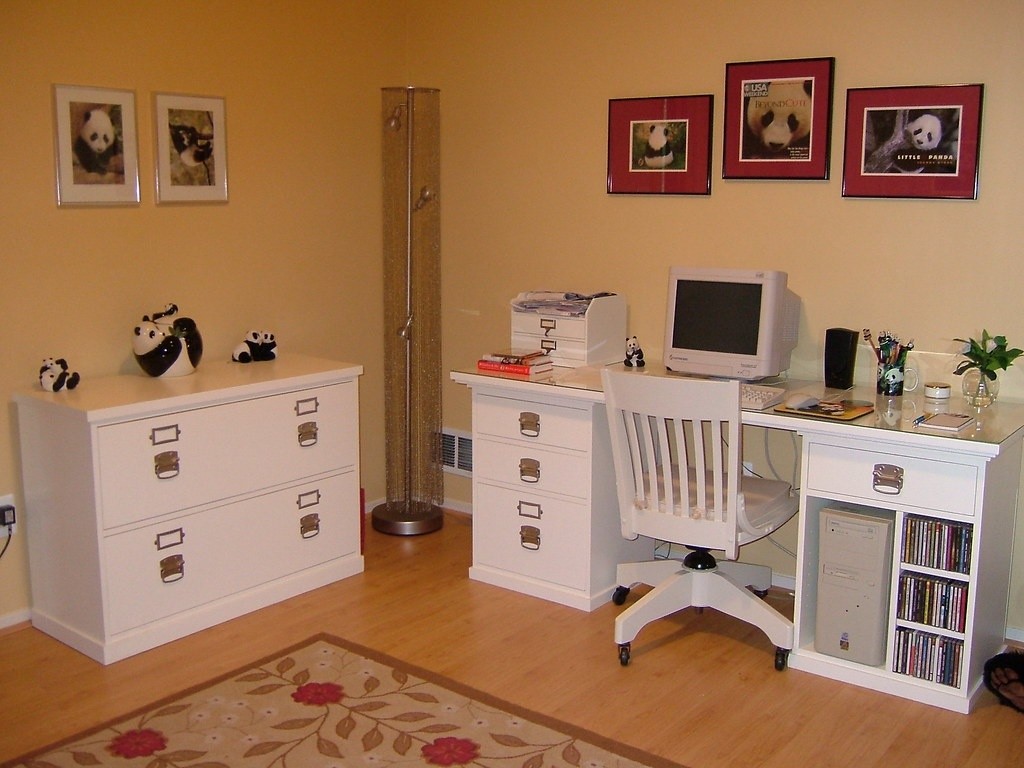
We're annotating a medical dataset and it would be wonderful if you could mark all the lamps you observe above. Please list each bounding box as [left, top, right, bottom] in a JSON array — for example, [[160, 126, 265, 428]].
[[371, 84, 445, 535]]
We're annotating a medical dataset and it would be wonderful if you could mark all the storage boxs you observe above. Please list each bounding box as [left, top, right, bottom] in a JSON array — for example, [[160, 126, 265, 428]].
[[511, 292, 626, 369]]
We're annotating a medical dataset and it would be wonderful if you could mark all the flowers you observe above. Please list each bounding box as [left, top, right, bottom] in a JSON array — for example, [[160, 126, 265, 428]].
[[952, 329, 1024, 381]]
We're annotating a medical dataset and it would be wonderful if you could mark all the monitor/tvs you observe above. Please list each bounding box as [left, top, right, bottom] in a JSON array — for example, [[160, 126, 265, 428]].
[[663, 265, 801, 380]]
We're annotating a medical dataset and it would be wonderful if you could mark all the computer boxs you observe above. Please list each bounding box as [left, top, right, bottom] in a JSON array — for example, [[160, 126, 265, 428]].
[[815, 501, 897, 668]]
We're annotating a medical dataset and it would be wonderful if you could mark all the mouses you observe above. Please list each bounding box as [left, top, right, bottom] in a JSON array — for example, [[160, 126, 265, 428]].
[[785, 392, 820, 409]]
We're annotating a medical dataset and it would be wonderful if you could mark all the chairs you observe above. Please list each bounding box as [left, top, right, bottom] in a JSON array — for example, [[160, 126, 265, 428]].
[[600, 366, 795, 671]]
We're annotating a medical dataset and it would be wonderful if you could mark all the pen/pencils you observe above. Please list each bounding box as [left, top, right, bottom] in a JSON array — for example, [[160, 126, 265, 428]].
[[863, 327, 912, 364], [911, 413, 932, 426]]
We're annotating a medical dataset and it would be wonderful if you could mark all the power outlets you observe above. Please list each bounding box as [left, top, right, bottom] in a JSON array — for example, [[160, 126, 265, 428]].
[[0, 493, 17, 537]]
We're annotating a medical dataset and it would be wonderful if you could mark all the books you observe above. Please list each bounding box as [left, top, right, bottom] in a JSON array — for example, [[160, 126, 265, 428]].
[[898, 570, 969, 634], [477, 348, 554, 375], [920, 413, 976, 432], [901, 513, 974, 575], [892, 626, 964, 689]]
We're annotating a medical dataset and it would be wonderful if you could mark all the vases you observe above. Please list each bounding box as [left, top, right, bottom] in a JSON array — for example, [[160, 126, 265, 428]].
[[962, 370, 999, 408]]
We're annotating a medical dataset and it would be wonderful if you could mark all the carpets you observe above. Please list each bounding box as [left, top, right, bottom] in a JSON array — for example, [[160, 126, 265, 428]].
[[0, 633, 682, 768]]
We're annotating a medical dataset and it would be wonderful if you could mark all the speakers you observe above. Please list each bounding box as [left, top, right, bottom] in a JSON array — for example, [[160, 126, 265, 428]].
[[826, 328, 860, 389]]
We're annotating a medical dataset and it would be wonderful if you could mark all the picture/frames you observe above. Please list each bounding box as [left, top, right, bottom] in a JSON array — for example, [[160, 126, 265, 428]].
[[721, 57, 835, 180], [841, 82, 984, 200], [151, 89, 230, 206], [607, 94, 714, 195], [51, 83, 141, 209]]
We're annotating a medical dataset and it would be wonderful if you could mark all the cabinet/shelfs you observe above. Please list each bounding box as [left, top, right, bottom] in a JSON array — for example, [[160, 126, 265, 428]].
[[448, 361, 1024, 715], [14, 354, 364, 665]]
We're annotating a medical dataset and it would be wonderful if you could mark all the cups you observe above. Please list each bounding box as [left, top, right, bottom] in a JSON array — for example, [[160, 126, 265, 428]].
[[877, 363, 918, 396]]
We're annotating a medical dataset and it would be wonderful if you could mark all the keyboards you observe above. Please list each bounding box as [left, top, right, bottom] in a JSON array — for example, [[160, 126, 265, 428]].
[[738, 383, 786, 410]]
